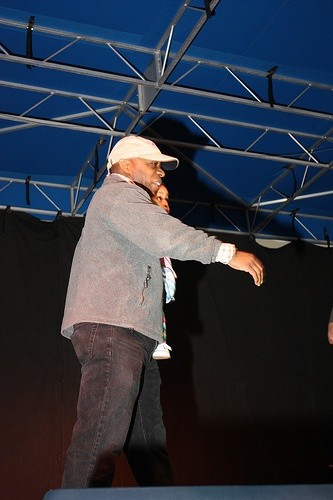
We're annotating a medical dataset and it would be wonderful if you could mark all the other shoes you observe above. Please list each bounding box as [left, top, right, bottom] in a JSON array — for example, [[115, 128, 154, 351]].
[[153, 343, 172, 359]]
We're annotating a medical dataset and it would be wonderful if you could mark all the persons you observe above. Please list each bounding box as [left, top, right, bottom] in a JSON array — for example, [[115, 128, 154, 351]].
[[59, 134, 265, 489]]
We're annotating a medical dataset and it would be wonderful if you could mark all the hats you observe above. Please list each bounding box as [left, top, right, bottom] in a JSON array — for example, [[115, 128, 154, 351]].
[[106, 136, 179, 176]]
